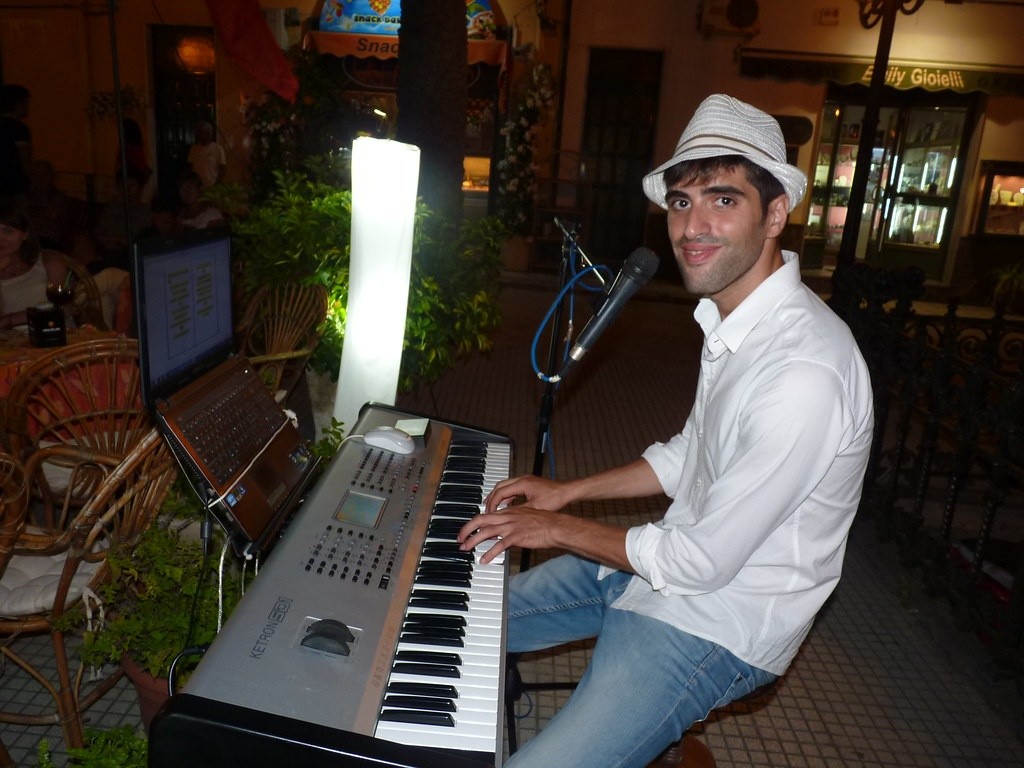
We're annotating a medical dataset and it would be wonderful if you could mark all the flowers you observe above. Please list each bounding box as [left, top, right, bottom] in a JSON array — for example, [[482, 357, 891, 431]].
[[494, 62, 559, 237], [83, 83, 149, 123]]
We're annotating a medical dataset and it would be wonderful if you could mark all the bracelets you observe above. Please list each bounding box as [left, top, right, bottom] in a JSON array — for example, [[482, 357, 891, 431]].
[[9, 314, 12, 327]]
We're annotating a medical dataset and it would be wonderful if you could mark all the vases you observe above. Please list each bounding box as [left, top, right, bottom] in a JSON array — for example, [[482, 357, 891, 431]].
[[496, 237, 534, 272]]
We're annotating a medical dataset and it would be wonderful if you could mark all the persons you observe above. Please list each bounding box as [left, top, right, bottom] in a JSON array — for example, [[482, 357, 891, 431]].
[[178, 172, 220, 228], [0, 84, 32, 214], [117, 118, 145, 172], [0, 208, 68, 341], [188, 122, 225, 187], [457, 95, 874, 768]]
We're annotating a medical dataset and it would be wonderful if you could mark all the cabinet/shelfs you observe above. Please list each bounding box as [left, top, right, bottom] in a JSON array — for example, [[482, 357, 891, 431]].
[[526, 172, 593, 274], [969, 160, 1024, 240], [803, 106, 966, 251]]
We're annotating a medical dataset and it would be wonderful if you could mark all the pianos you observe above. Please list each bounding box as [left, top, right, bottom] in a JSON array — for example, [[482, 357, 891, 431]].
[[145, 403, 517, 768]]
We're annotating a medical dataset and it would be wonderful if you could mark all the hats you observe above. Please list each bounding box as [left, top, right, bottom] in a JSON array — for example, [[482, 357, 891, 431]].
[[642, 94, 807, 213]]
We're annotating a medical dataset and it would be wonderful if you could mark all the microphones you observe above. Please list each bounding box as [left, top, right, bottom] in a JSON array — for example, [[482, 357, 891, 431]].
[[560, 247, 661, 378]]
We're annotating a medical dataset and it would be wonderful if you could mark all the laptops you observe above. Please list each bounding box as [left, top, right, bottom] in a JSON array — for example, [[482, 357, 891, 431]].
[[129, 226, 314, 554]]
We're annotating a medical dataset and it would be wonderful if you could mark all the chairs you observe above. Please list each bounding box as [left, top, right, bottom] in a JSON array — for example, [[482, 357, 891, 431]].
[[0, 249, 328, 768]]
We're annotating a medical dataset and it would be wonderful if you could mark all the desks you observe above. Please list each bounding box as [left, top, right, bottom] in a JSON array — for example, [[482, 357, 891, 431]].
[[0, 323, 151, 444]]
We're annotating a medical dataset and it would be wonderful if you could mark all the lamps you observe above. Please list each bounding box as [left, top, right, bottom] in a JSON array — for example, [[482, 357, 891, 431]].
[[332, 136, 422, 436]]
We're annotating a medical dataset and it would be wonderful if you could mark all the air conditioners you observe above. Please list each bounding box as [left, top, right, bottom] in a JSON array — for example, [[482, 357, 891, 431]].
[[698, 0, 762, 42]]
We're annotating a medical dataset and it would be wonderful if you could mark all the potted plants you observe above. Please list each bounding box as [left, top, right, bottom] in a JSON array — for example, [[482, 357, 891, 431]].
[[45, 416, 345, 737]]
